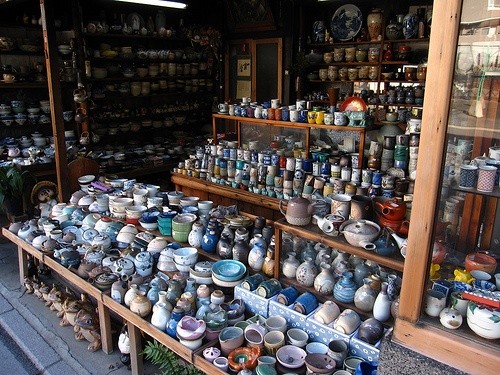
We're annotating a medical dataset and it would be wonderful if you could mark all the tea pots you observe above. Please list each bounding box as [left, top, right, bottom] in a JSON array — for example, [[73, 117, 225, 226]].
[[113, 257, 135, 282], [74, 107, 89, 124], [277, 193, 450, 278], [67, 261, 97, 278], [91, 128, 100, 143], [79, 131, 90, 146], [72, 88, 89, 104], [453, 267, 477, 285], [87, 272, 118, 290], [84, 264, 111, 278]]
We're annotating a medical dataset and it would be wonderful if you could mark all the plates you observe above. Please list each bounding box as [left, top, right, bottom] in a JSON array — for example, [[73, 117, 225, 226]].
[[126, 13, 145, 35], [330, 4, 363, 41]]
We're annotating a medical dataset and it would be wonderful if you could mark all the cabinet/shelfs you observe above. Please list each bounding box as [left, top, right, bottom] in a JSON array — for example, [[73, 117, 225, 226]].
[[0, 0, 500, 375]]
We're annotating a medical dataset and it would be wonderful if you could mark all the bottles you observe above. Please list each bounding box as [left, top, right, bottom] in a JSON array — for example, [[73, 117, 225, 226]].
[[23, 9, 130, 35], [311, 20, 330, 43], [386, 8, 432, 40], [112, 215, 402, 344], [146, 10, 166, 35], [367, 8, 385, 43]]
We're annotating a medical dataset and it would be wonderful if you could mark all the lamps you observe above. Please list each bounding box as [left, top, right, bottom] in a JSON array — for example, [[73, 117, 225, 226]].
[[116, 0, 189, 9]]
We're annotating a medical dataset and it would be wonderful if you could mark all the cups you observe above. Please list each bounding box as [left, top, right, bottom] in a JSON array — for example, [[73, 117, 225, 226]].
[[173, 43, 426, 199], [439, 136, 500, 237], [212, 357, 229, 373]]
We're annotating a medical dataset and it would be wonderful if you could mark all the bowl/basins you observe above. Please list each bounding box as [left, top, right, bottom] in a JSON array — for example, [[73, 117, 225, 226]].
[[0, 35, 224, 171], [424, 250, 500, 341], [257, 315, 366, 375], [9, 173, 253, 352]]
